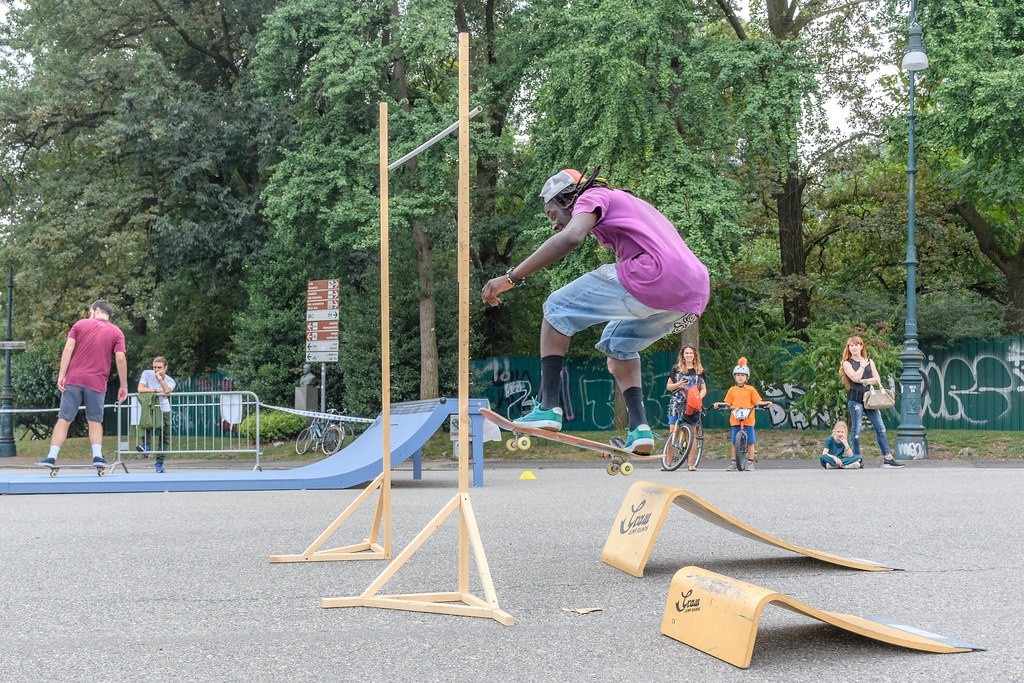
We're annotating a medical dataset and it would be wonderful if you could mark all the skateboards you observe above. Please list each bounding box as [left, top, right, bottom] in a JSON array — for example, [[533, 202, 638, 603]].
[[41, 460, 113, 478], [478, 407, 668, 476]]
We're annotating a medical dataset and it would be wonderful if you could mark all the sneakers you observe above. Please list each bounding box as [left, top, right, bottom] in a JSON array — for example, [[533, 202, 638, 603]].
[[745, 462, 755, 471], [622, 423, 655, 457], [93, 455, 107, 468], [725, 464, 737, 471], [136, 445, 151, 459], [155, 462, 165, 473], [512, 395, 563, 432], [860, 461, 865, 468], [883, 457, 904, 468], [35, 458, 55, 468]]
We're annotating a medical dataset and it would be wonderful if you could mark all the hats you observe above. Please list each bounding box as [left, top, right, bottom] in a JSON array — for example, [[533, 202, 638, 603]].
[[539, 169, 609, 205]]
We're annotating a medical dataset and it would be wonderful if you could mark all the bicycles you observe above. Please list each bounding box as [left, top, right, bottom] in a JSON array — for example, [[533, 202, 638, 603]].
[[717, 405, 767, 472], [294, 408, 347, 456], [661, 388, 713, 471]]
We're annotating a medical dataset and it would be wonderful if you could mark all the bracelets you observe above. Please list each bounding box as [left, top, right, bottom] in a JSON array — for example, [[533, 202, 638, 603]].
[[120, 386, 127, 388], [860, 365, 865, 369], [844, 446, 850, 452], [506, 267, 525, 288]]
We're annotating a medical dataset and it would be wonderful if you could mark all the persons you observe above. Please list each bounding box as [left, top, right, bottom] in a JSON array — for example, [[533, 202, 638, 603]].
[[820, 421, 862, 469], [713, 357, 772, 471], [479, 167, 711, 455], [38, 299, 128, 467], [661, 345, 707, 471], [135, 356, 177, 473], [838, 336, 906, 468]]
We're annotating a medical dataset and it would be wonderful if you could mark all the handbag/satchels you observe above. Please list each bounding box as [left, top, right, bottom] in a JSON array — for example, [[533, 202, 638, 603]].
[[862, 378, 896, 409]]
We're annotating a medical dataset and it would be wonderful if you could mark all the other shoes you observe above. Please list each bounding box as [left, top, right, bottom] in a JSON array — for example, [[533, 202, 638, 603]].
[[845, 461, 860, 469], [661, 463, 673, 471], [825, 462, 837, 469], [687, 464, 696, 471]]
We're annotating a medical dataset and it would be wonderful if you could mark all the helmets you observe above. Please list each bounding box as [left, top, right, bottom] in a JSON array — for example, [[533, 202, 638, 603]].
[[732, 364, 750, 382]]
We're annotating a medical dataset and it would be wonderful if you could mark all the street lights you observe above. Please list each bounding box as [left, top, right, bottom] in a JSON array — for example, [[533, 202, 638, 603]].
[[890, 22, 930, 460]]
